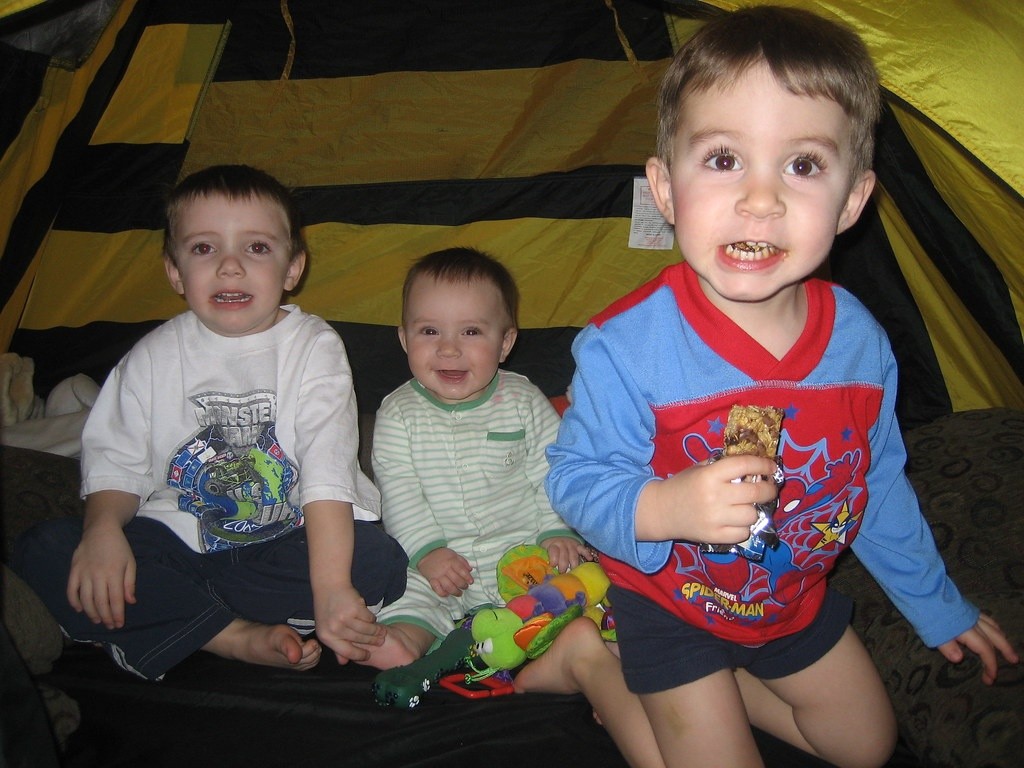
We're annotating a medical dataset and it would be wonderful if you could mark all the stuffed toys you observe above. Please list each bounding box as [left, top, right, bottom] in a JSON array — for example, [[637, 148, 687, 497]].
[[466, 543, 617, 684], [372, 628, 489, 710]]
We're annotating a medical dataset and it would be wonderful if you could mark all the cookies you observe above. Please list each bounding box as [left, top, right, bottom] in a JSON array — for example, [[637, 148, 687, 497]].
[[723, 403, 785, 461]]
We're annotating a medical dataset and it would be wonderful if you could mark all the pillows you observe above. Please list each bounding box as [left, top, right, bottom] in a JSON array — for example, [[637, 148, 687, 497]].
[[0, 444, 85, 566], [829, 406, 1024, 768]]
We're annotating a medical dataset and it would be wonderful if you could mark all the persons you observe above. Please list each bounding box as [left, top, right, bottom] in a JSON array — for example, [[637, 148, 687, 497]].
[[512, 6, 1019, 768], [351, 246, 593, 672], [11, 165, 410, 674]]
